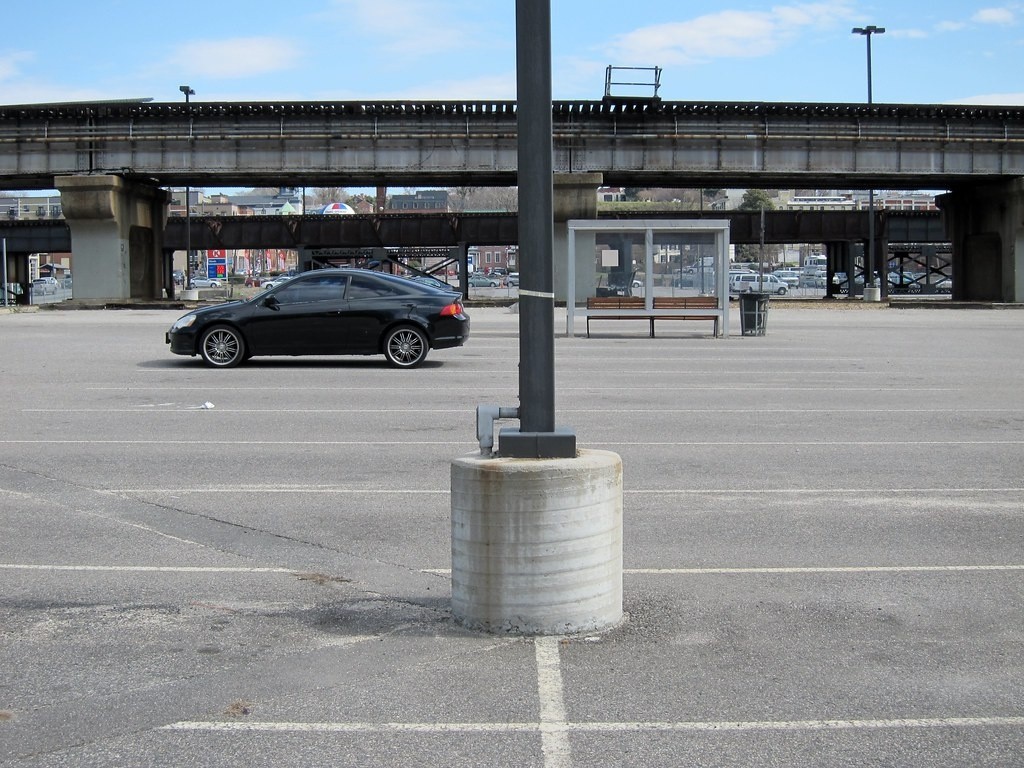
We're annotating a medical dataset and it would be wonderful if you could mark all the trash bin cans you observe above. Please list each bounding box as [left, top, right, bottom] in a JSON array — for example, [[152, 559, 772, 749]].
[[739, 292, 770, 332]]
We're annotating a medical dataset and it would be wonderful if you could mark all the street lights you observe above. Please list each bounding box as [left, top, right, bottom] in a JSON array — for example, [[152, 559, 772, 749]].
[[757, 200, 767, 293], [179, 84, 197, 291], [851, 25, 886, 288]]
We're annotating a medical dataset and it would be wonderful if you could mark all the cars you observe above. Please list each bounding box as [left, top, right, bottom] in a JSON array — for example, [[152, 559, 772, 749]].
[[728, 253, 952, 295], [173, 269, 185, 286], [503, 272, 520, 287], [30, 276, 72, 297], [245, 276, 270, 288], [184, 275, 222, 289], [164, 267, 473, 369], [631, 279, 644, 288], [260, 277, 305, 292], [457, 267, 507, 280], [683, 260, 713, 273], [468, 275, 500, 288]]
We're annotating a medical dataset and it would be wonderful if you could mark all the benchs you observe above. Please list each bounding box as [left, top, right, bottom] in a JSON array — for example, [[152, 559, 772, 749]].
[[586, 297, 651, 338], [652, 297, 718, 339]]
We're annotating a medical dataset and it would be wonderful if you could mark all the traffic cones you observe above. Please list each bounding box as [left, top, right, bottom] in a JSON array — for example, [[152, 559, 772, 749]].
[[500, 279, 505, 288]]
